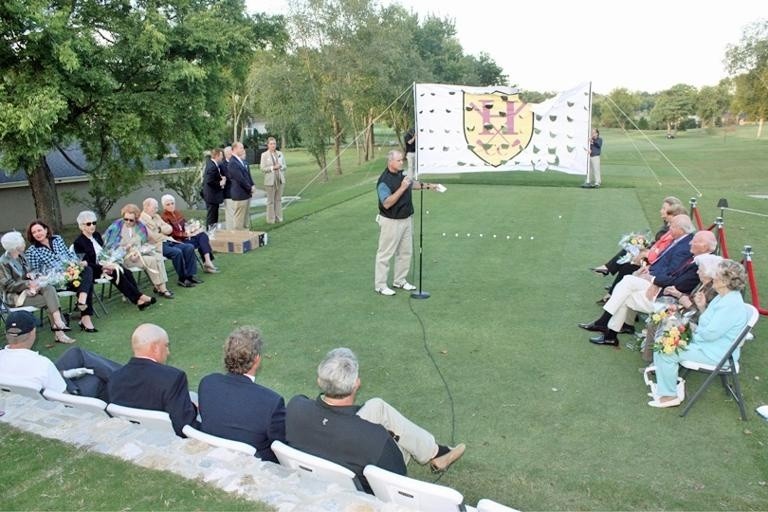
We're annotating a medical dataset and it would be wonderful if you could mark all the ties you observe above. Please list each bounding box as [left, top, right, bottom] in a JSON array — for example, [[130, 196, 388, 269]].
[[687, 256, 694, 263]]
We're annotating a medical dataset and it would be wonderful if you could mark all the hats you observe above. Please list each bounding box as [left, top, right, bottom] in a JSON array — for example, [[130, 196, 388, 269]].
[[6, 310, 41, 337]]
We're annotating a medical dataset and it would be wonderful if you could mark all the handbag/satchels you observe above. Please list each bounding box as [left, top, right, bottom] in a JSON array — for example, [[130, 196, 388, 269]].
[[644, 366, 685, 403]]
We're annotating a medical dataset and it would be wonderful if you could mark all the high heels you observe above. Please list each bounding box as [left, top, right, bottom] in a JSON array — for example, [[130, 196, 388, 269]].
[[588, 266, 609, 277]]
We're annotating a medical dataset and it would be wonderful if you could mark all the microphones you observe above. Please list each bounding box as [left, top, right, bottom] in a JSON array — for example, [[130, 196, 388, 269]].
[[401, 171, 408, 178]]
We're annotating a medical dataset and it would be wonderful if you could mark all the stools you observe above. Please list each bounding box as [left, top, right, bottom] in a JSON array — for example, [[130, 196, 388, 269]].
[[195, 251, 203, 271], [56, 291, 77, 313], [0, 292, 48, 326]]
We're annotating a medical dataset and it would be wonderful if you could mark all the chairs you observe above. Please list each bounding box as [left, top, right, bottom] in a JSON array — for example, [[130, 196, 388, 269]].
[[69, 244, 111, 318], [40, 388, 107, 412], [271, 440, 364, 492], [101, 235, 145, 302], [476, 499, 522, 512], [182, 425, 261, 459], [0, 380, 45, 400], [363, 464, 476, 512], [107, 403, 176, 434], [677, 303, 760, 421]]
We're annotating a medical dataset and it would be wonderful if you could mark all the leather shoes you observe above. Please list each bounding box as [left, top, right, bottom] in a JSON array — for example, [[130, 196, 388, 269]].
[[78, 319, 98, 333], [158, 290, 174, 298], [177, 279, 195, 288], [617, 325, 635, 335], [203, 262, 219, 270], [430, 442, 466, 475], [589, 333, 619, 346], [203, 267, 221, 274], [648, 396, 681, 408], [74, 300, 88, 312], [138, 302, 151, 311], [188, 275, 204, 283], [604, 284, 612, 290], [596, 295, 611, 305], [153, 286, 172, 293], [147, 296, 157, 304], [577, 320, 608, 333]]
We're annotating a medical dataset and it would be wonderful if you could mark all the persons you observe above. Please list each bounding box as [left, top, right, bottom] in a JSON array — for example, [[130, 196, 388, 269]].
[[404, 129, 416, 180], [198, 325, 285, 463], [590, 129, 602, 187], [0, 310, 124, 397], [374, 149, 440, 295], [203, 142, 255, 230], [260, 136, 286, 223], [284, 346, 467, 497], [578, 196, 748, 408], [105, 323, 201, 438], [1, 194, 219, 344]]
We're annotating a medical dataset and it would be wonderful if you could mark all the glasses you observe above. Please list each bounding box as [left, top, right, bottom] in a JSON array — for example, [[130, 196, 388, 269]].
[[82, 221, 96, 226], [123, 219, 134, 222]]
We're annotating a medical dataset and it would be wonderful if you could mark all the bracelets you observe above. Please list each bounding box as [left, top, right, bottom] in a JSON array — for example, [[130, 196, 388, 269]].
[[426, 183, 432, 189]]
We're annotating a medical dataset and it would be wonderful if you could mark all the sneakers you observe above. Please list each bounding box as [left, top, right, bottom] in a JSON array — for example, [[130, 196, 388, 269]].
[[375, 287, 396, 296], [393, 282, 417, 290], [638, 361, 654, 375]]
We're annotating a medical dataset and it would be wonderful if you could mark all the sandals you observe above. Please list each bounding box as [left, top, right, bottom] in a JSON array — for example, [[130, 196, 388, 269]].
[[54, 333, 76, 344], [51, 322, 72, 331]]
